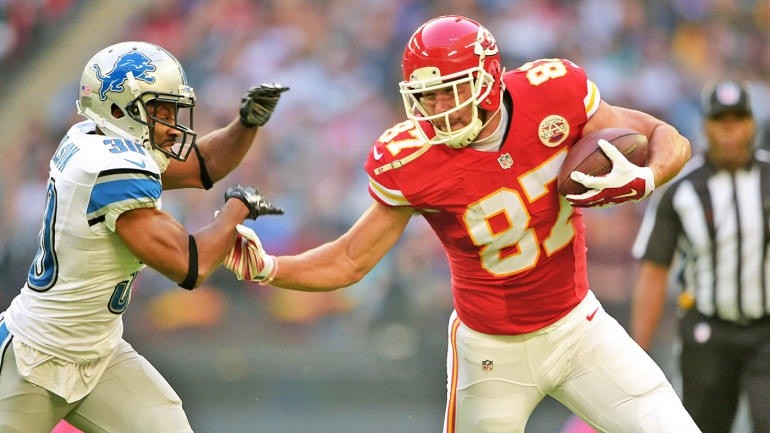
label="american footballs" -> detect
[556,128,648,194]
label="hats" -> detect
[703,81,752,117]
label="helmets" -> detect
[401,14,505,111]
[76,40,190,174]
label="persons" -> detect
[0,40,291,433]
[622,77,769,433]
[0,0,770,329]
[215,13,706,433]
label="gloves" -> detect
[566,138,654,208]
[224,182,285,220]
[213,210,278,285]
[240,82,290,127]
